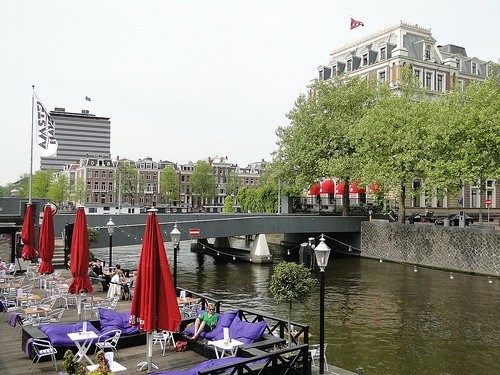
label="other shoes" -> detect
[190,335,199,340]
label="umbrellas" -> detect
[39,208,55,291]
[68,207,93,320]
[128,208,182,373]
[21,205,36,272]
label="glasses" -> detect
[209,308,215,311]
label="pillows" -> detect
[97,306,141,337]
[229,315,271,344]
[182,308,240,338]
[38,319,110,350]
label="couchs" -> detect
[173,316,288,358]
[146,345,292,375]
[21,318,146,364]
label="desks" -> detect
[177,296,202,308]
[13,294,41,306]
[123,278,131,284]
[45,277,66,295]
[102,270,114,282]
[6,275,15,282]
[209,337,245,360]
[87,360,127,375]
[67,330,99,365]
[21,308,50,322]
[86,296,106,311]
[0,282,23,293]
[57,285,68,295]
[285,330,313,346]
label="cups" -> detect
[180,295,183,300]
[224,341,229,347]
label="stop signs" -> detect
[485,198,491,205]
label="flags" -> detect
[350,18,364,29]
[86,96,92,101]
[33,91,58,157]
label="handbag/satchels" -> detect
[175,341,187,352]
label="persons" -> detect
[0,259,8,274]
[190,304,218,341]
[88,262,137,299]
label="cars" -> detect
[434,213,474,226]
[408,211,437,225]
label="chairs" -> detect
[309,342,329,372]
[0,262,200,375]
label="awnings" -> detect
[309,179,385,195]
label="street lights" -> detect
[106,215,116,273]
[170,221,181,298]
[314,235,332,375]
